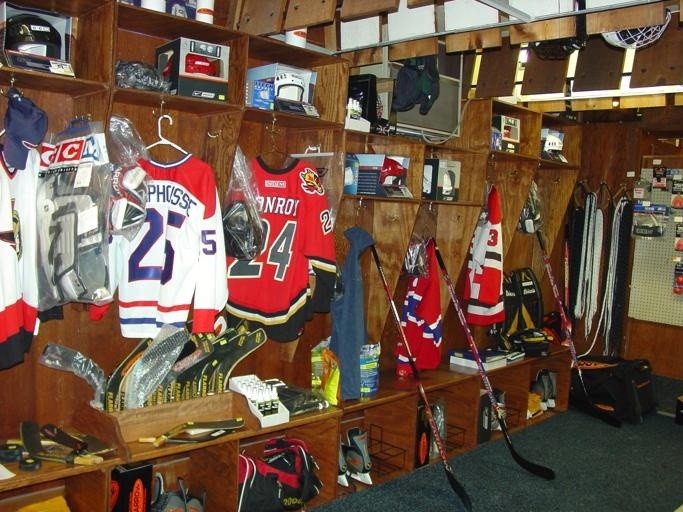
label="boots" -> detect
[344,428,371,472]
[335,435,347,474]
[529,370,556,402]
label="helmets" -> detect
[0,13,61,73]
[601,10,672,50]
[530,36,585,62]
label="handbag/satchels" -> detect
[237,435,325,512]
[571,354,659,428]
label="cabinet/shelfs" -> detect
[0,0,584,512]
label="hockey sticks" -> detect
[371,245,472,512]
[536,230,623,428]
[432,240,554,480]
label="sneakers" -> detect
[152,477,207,512]
